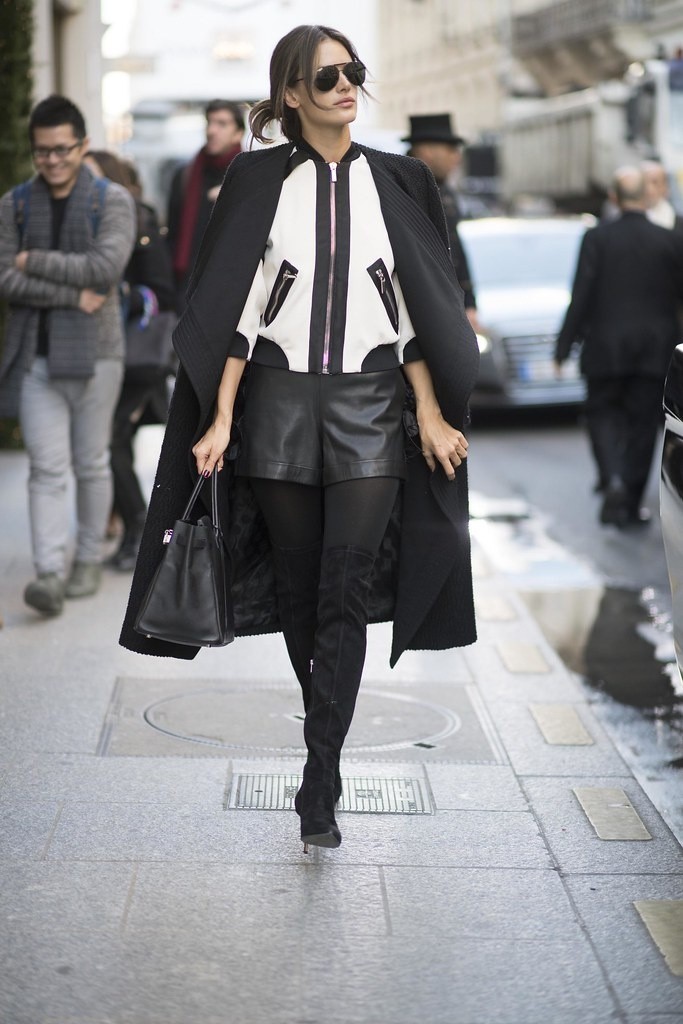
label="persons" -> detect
[166,98,249,317]
[0,92,138,615]
[190,22,471,854]
[553,156,683,529]
[76,149,176,573]
[403,110,476,324]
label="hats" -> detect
[399,114,463,145]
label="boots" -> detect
[294,545,376,850]
[272,549,342,805]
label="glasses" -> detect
[290,60,366,91]
[31,140,83,158]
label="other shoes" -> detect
[24,572,65,616]
[113,510,148,572]
[64,561,101,597]
[628,507,651,529]
[597,476,626,524]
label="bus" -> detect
[493,57,682,231]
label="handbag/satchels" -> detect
[132,461,234,647]
[121,284,178,381]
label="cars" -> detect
[456,213,594,411]
[658,344,683,679]
